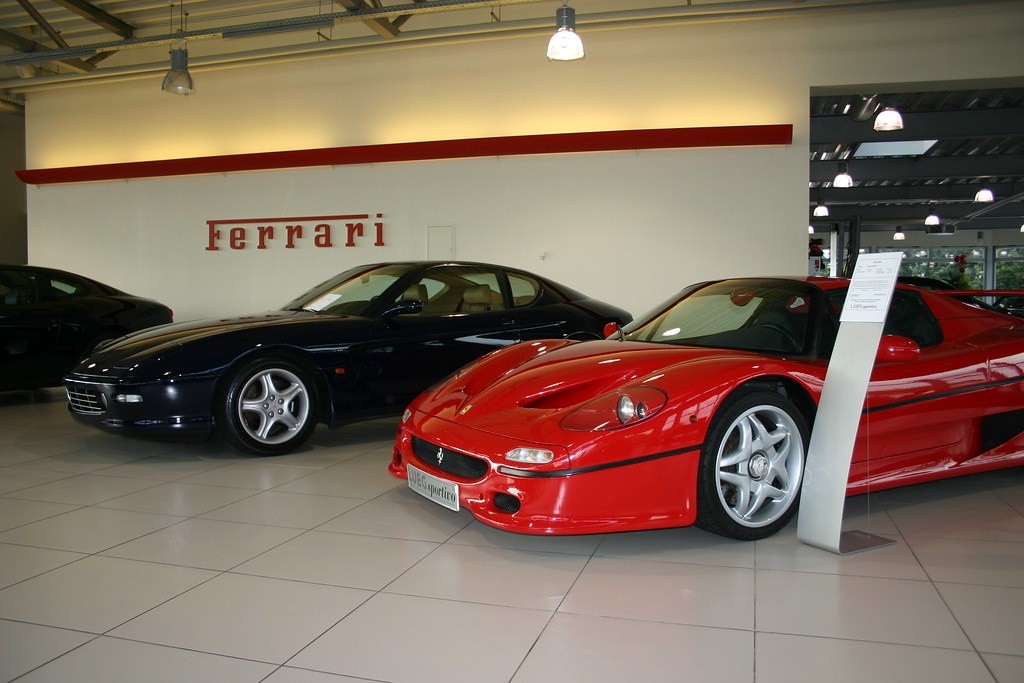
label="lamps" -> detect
[873,95,904,133]
[1019,224,1024,233]
[545,0,585,61]
[924,205,941,225]
[925,226,955,236]
[893,226,906,241]
[832,162,854,188]
[809,224,815,235]
[813,200,829,218]
[974,181,995,202]
[161,0,197,97]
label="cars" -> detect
[992,294,1024,319]
[0,264,174,391]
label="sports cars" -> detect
[388,276,1024,541]
[63,260,636,455]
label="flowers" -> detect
[936,254,975,290]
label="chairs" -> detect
[883,297,937,347]
[397,284,433,316]
[462,284,492,313]
